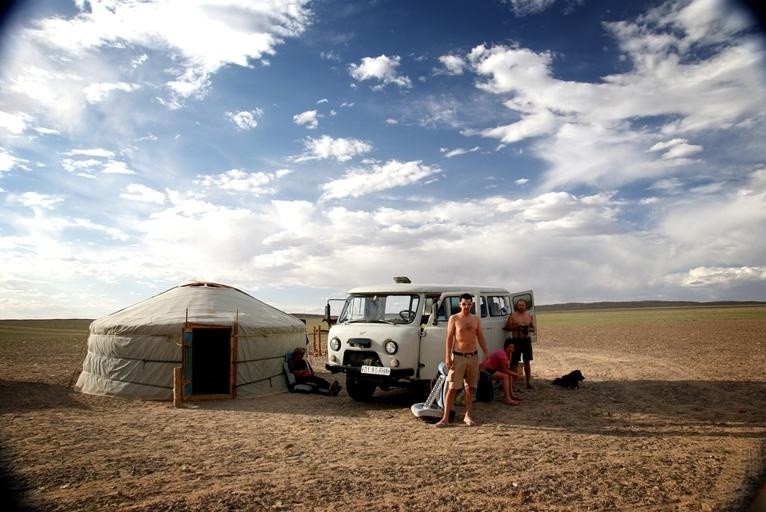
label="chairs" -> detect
[410,361,456,422]
[284,352,319,393]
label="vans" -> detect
[321,276,538,402]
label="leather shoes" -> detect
[331,381,339,393]
[333,385,342,396]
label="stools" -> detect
[475,378,496,403]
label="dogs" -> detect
[550,369,586,390]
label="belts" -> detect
[452,351,479,359]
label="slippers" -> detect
[513,396,524,400]
[505,401,519,406]
[463,419,476,426]
[436,419,448,427]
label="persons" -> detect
[479,338,527,405]
[435,294,490,428]
[287,348,342,396]
[505,299,537,392]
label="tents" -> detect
[71,281,308,400]
